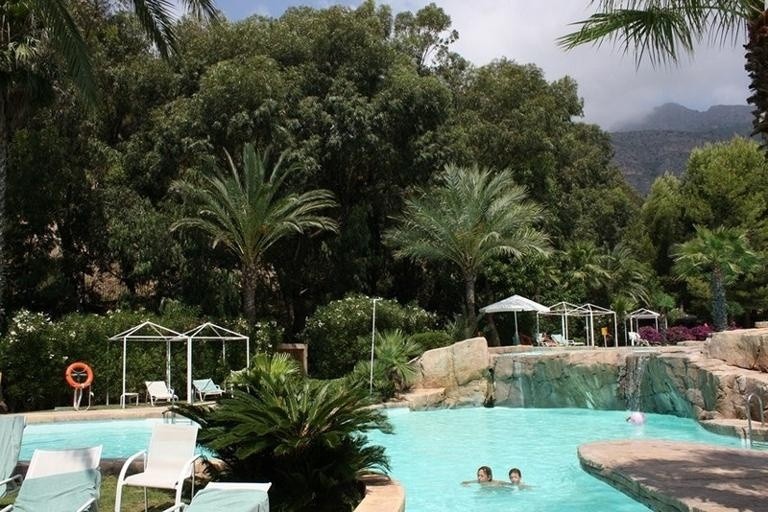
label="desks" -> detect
[120,392,139,406]
[230,383,251,398]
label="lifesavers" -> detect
[66,362,93,388]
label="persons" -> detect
[461,466,527,489]
[540,333,555,345]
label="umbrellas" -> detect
[479,294,550,342]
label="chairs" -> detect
[1,413,272,512]
[536,332,585,347]
[192,377,225,401]
[144,379,179,406]
[628,331,649,346]
